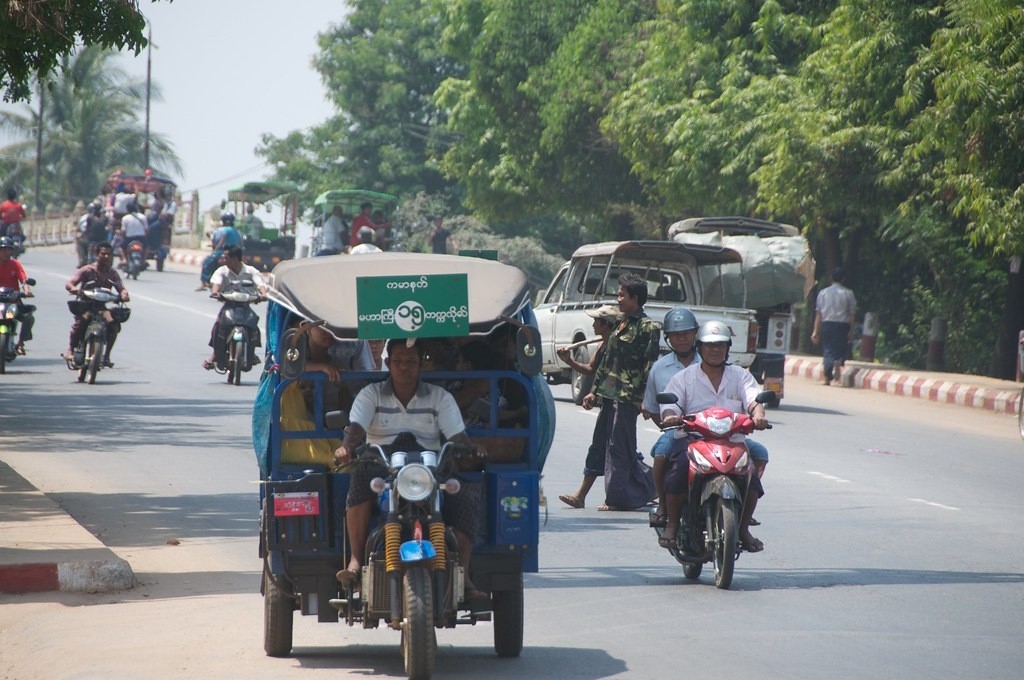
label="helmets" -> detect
[109,307,131,323]
[221,211,234,224]
[67,301,93,316]
[0,236,15,249]
[357,225,376,242]
[83,202,94,211]
[696,320,732,348]
[663,307,699,333]
[126,201,137,211]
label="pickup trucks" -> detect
[534,241,759,406]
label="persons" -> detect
[240,204,262,227]
[0,188,27,253]
[202,243,268,370]
[286,320,529,431]
[196,212,243,292]
[557,274,660,510]
[811,267,856,386]
[334,339,489,600]
[642,308,769,552]
[74,182,177,269]
[350,203,394,251]
[428,215,457,254]
[320,205,346,249]
[65,242,129,368]
[0,236,34,355]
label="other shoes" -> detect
[597,504,622,511]
[833,359,841,382]
[822,379,830,385]
[559,495,585,509]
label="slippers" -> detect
[750,517,760,527]
[202,359,213,370]
[466,587,488,600]
[742,535,764,552]
[651,506,666,526]
[659,525,677,547]
[336,568,362,593]
[15,344,26,356]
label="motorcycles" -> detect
[5,204,28,260]
[658,391,777,588]
[258,255,556,680]
[62,278,129,384]
[105,173,177,272]
[308,189,398,255]
[205,292,267,385]
[0,277,36,374]
[205,181,296,281]
[116,231,144,280]
[74,220,99,264]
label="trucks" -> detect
[669,218,794,408]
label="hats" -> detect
[585,305,621,325]
